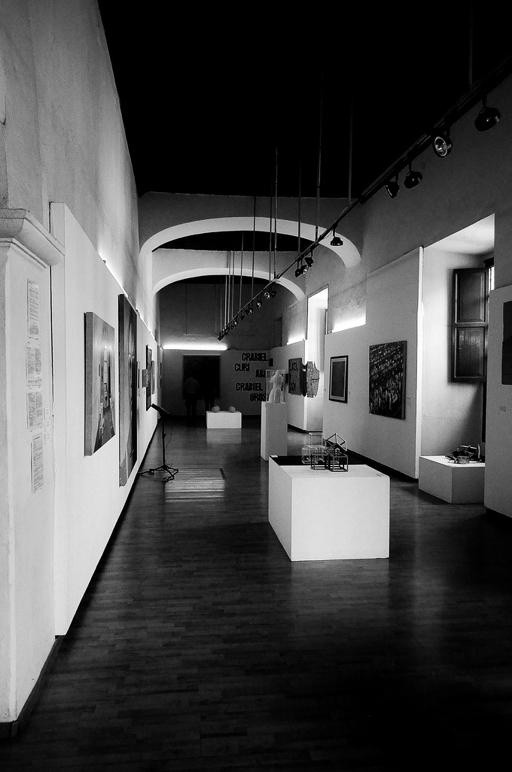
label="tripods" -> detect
[150,417,178,478]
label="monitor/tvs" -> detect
[288,358,302,395]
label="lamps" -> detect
[385,90,503,200]
[216,287,276,343]
[293,223,344,279]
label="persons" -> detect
[182,371,201,429]
[266,369,285,404]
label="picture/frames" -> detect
[328,355,348,403]
